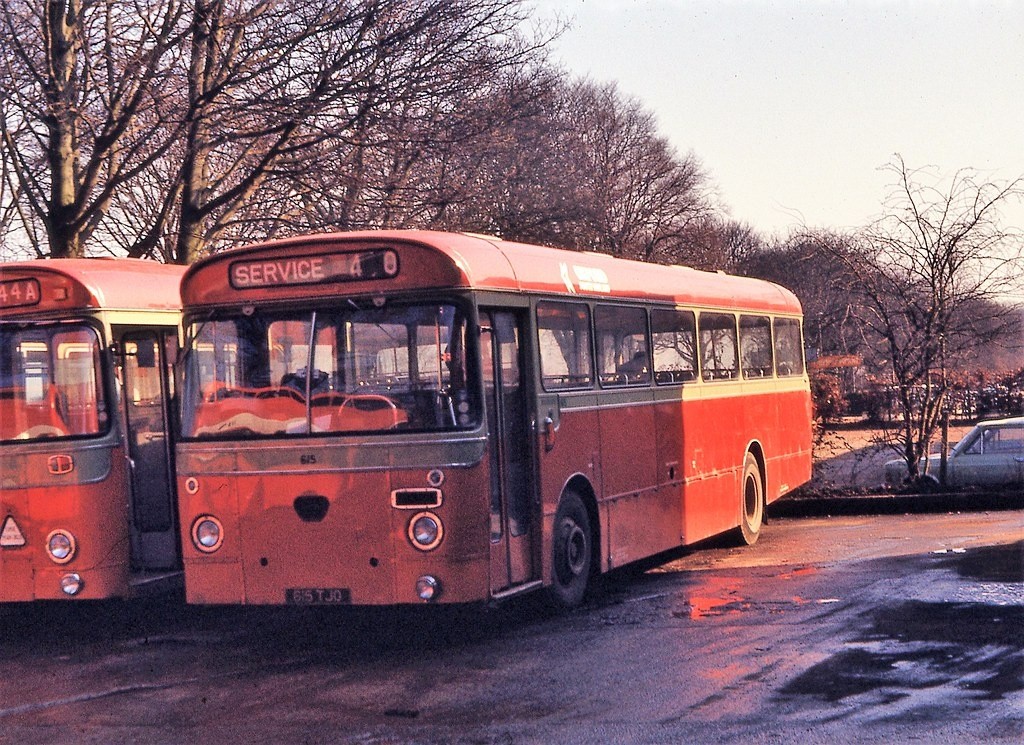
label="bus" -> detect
[0,256,617,610]
[175,229,816,618]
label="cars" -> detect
[885,416,1024,493]
